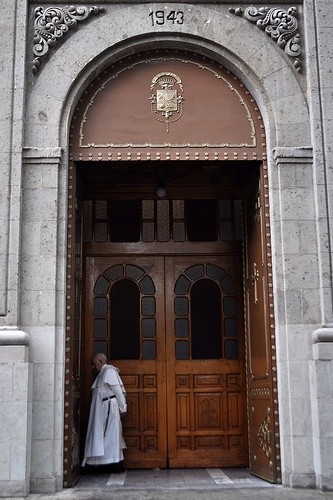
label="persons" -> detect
[81,353,129,474]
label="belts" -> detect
[102,395,115,402]
